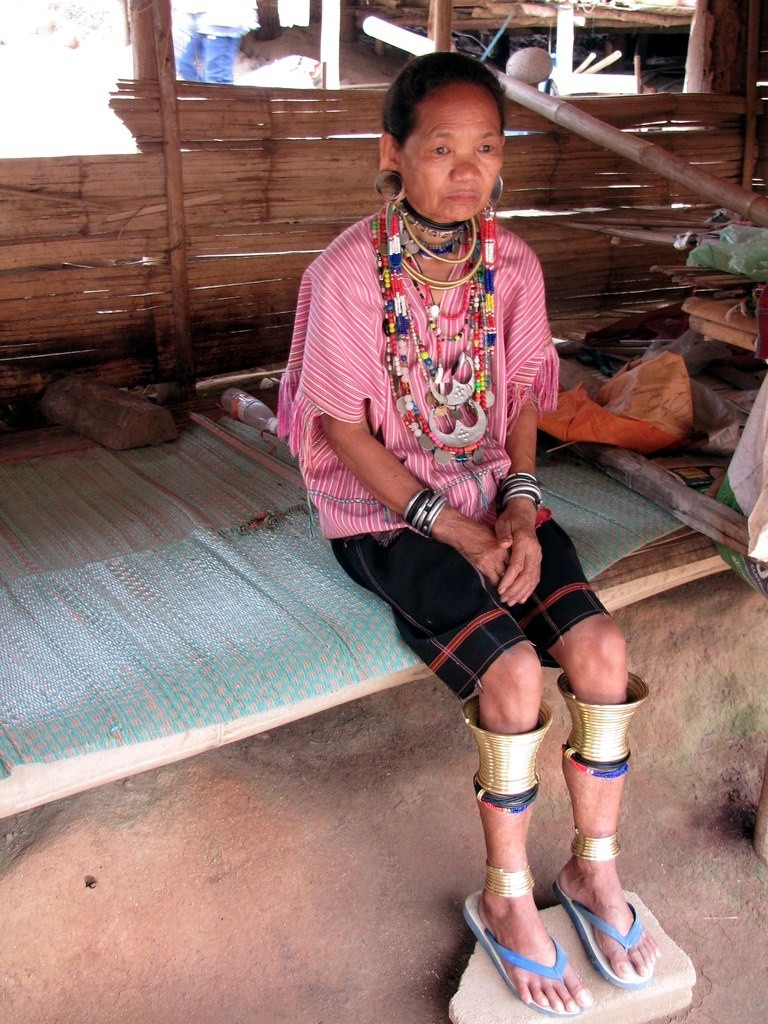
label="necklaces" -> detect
[369,186,502,467]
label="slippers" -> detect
[463,889,585,1018]
[553,878,656,990]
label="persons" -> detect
[177,0,261,87]
[274,47,667,1020]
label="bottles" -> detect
[221,387,278,434]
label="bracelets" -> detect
[497,470,544,512]
[401,486,449,539]
